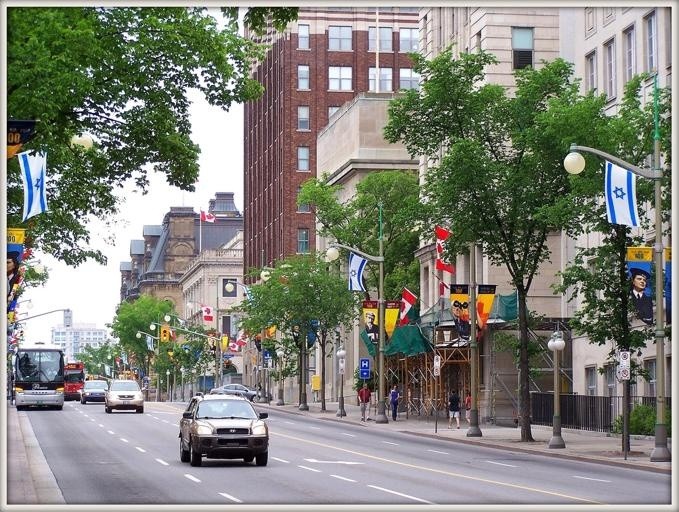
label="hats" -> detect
[366,313,375,319]
[630,268,650,279]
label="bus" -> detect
[62,360,85,401]
[15,341,65,411]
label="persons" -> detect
[366,312,388,345]
[389,385,399,420]
[447,389,461,429]
[465,393,471,423]
[358,383,372,421]
[7,251,20,294]
[630,268,652,323]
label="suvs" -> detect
[179,391,269,466]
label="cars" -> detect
[80,379,109,404]
[209,383,273,403]
[104,379,147,413]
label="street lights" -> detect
[97,313,208,403]
[28,115,102,158]
[260,264,308,412]
[546,328,566,450]
[275,349,284,407]
[326,204,389,425]
[7,298,32,403]
[335,343,346,419]
[226,278,260,404]
[564,75,671,464]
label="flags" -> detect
[17,149,48,224]
[292,320,318,349]
[146,335,155,352]
[605,160,641,227]
[348,252,368,292]
[435,225,454,273]
[202,209,215,224]
[399,287,418,326]
[450,283,497,337]
